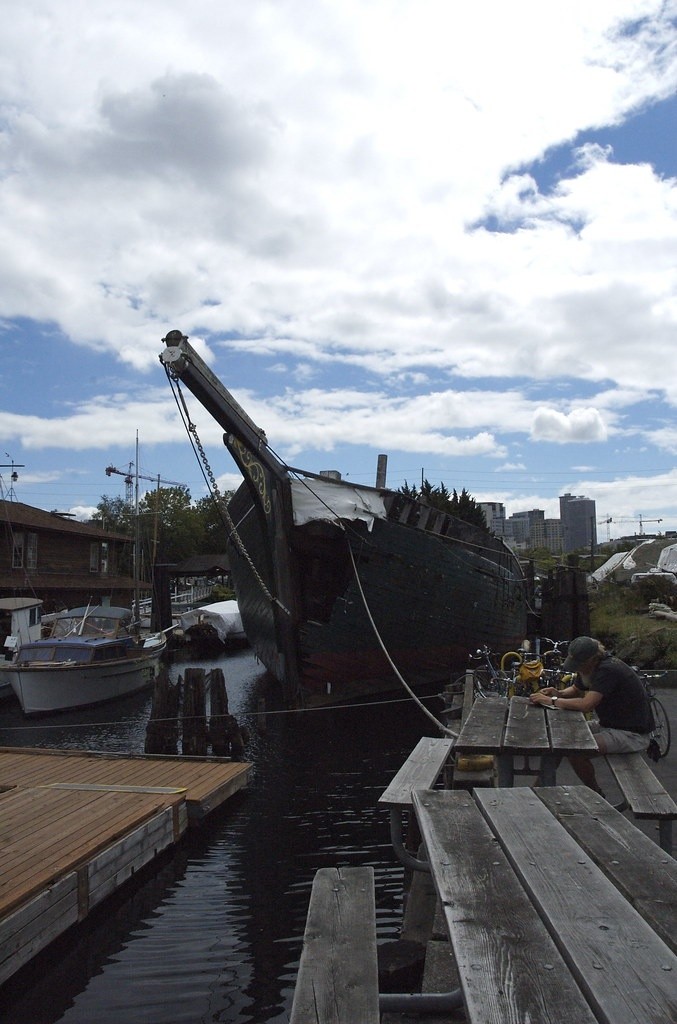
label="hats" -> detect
[563,636,598,671]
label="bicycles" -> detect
[467,638,670,759]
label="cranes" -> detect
[106,429,188,534]
[598,518,613,542]
[613,514,663,534]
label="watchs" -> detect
[551,696,557,707]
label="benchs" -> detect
[289,867,450,1024]
[376,736,455,873]
[604,753,677,856]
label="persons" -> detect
[529,636,656,799]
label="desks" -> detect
[410,783,677,1024]
[454,697,600,788]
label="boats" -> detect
[157,328,529,707]
[0,598,75,698]
[179,599,249,644]
[0,606,169,713]
